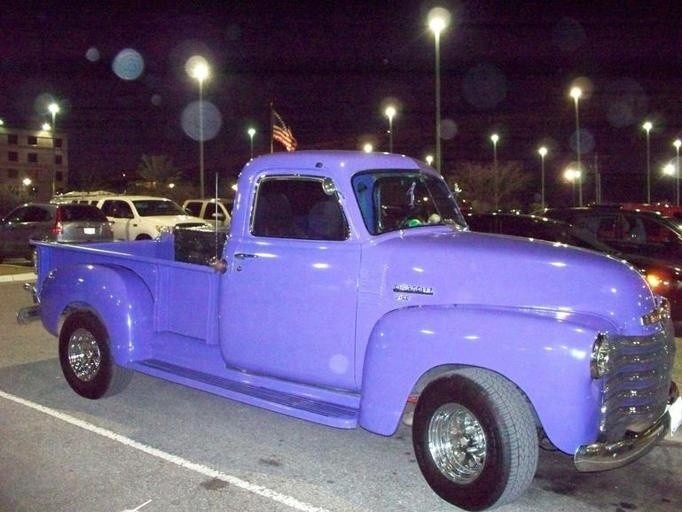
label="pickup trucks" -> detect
[15,150,682,512]
[55,195,213,241]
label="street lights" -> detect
[190,58,209,197]
[570,86,586,207]
[385,102,396,154]
[673,139,682,207]
[643,121,653,204]
[47,101,59,194]
[539,146,549,209]
[246,128,258,158]
[425,155,433,166]
[429,15,446,173]
[490,133,502,210]
[566,167,579,207]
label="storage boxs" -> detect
[171,221,226,270]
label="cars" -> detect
[0,201,114,265]
[461,197,682,327]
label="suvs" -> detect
[183,197,234,228]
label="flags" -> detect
[271,109,299,152]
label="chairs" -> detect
[255,193,307,239]
[143,202,162,217]
